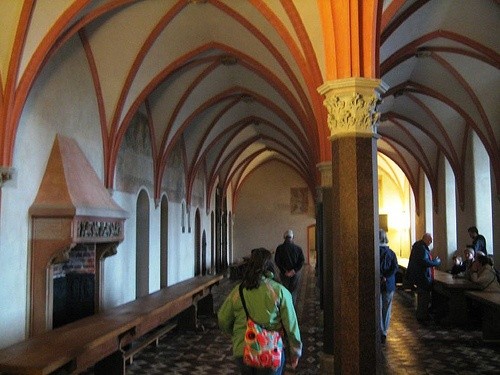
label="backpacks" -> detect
[496,270,500,284]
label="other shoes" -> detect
[381,334,386,344]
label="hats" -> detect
[283,229,294,239]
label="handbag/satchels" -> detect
[242,317,284,371]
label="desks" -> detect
[0,273,223,374]
[397,259,470,309]
[461,289,500,320]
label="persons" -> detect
[404,233,442,323]
[451,226,500,292]
[275,229,304,307]
[379,228,399,344]
[216,247,303,375]
